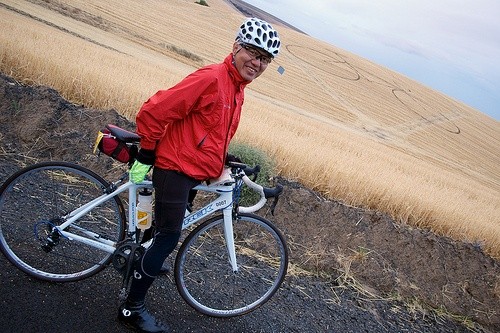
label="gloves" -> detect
[224,153,242,168]
[136,149,156,173]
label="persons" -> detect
[117,19,281,333]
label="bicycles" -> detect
[0,123,288,318]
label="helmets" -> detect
[235,17,281,59]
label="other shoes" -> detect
[159,263,171,276]
[117,304,167,333]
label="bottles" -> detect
[136,187,153,230]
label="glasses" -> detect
[240,44,272,64]
